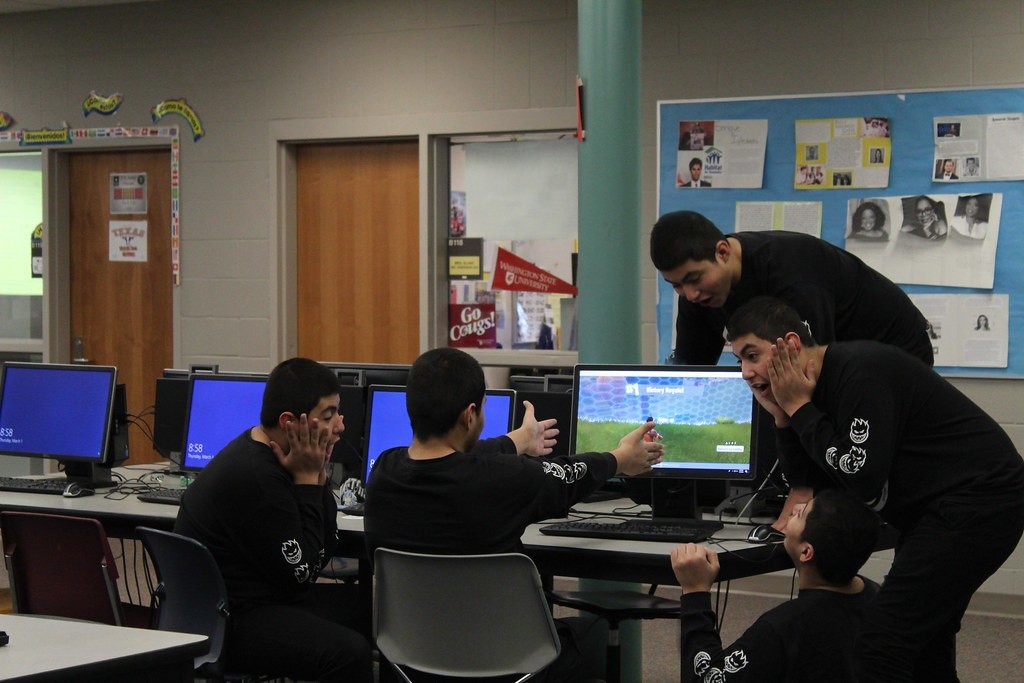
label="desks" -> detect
[0,613,209,683]
[0,463,896,642]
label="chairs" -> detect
[1,510,127,629]
[368,544,561,683]
[548,584,685,683]
[132,524,230,675]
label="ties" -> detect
[695,183,698,188]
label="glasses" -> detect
[915,207,934,217]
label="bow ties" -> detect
[945,173,951,177]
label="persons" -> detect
[935,159,958,179]
[901,197,946,240]
[949,124,960,136]
[873,149,884,163]
[680,157,712,187]
[796,167,824,185]
[952,196,989,238]
[646,416,663,442]
[649,210,931,372]
[963,158,980,176]
[974,314,991,332]
[724,297,1024,683]
[668,490,914,683]
[847,203,888,242]
[362,347,656,683]
[806,147,817,160]
[833,174,850,186]
[170,354,375,682]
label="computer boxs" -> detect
[58,383,130,465]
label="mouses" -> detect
[63,482,82,497]
[748,525,786,545]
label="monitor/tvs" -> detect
[0,361,118,491]
[153,364,759,521]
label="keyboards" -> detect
[540,521,724,544]
[0,476,75,495]
[138,491,185,506]
